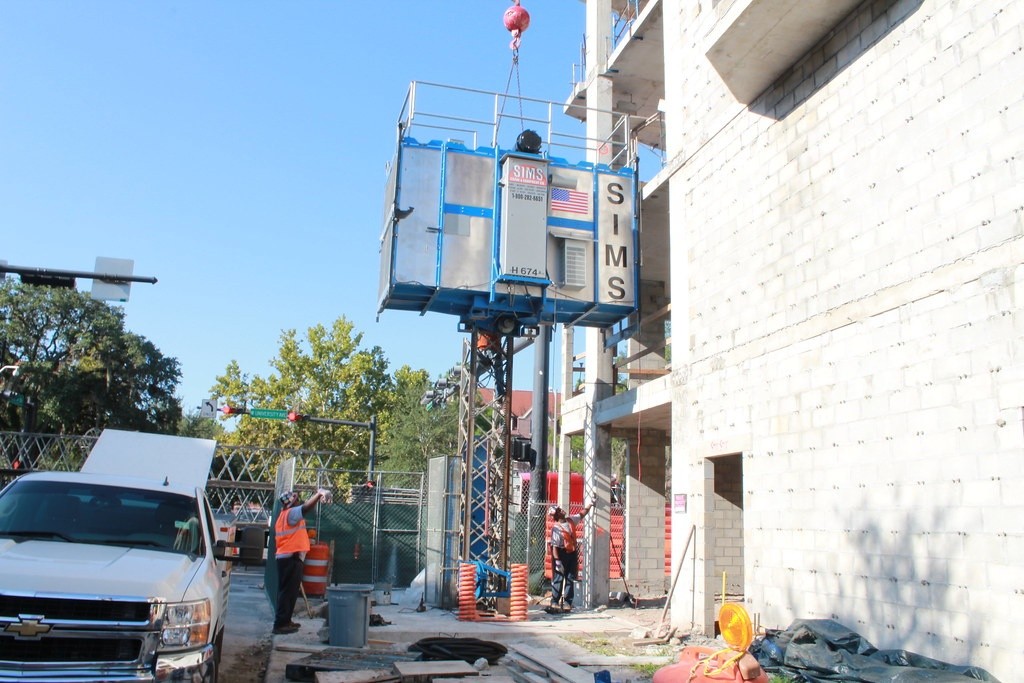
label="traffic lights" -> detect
[289,413,310,423]
[222,405,244,414]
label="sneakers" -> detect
[271,622,302,634]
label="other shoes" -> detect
[551,603,571,612]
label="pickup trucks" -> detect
[1,428,242,683]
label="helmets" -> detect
[548,505,560,518]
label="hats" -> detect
[278,491,297,509]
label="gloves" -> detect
[554,560,565,575]
[590,493,599,506]
[318,487,330,502]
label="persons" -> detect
[546,492,598,610]
[275,489,333,634]
